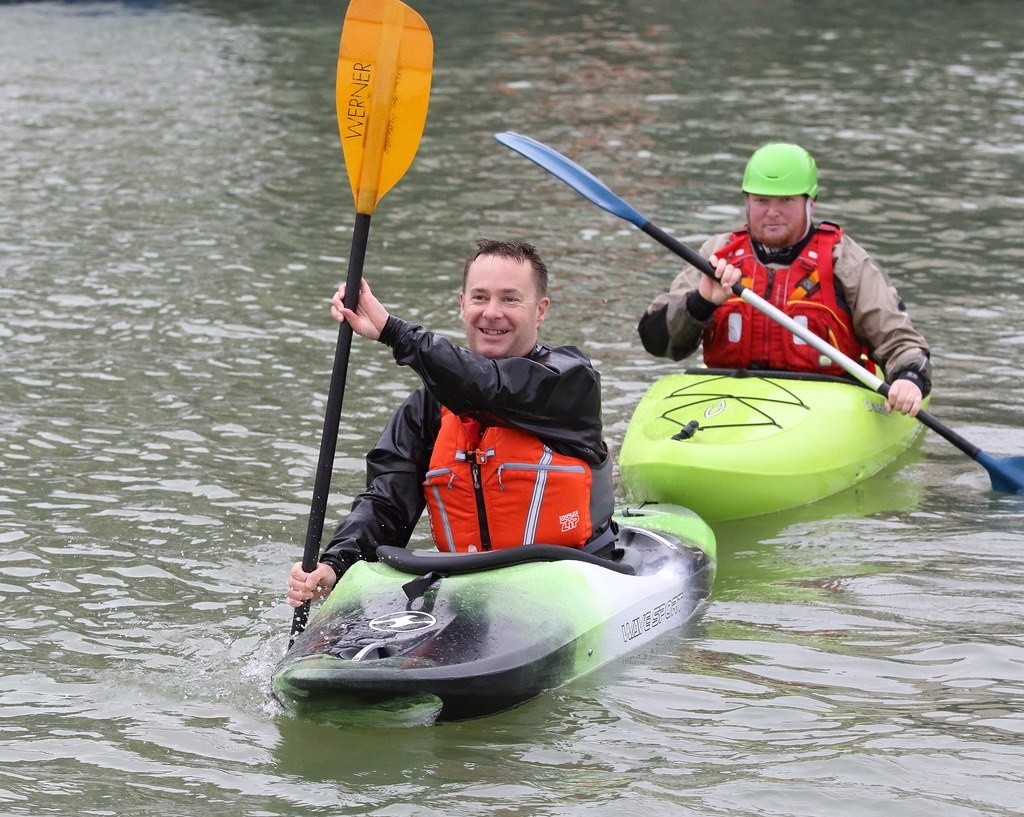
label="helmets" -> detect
[740,141,819,198]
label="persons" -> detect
[638,142,933,418]
[286,237,618,608]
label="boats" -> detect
[269,502,716,724]
[619,366,935,523]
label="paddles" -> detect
[495,131,1023,496]
[287,0,435,654]
